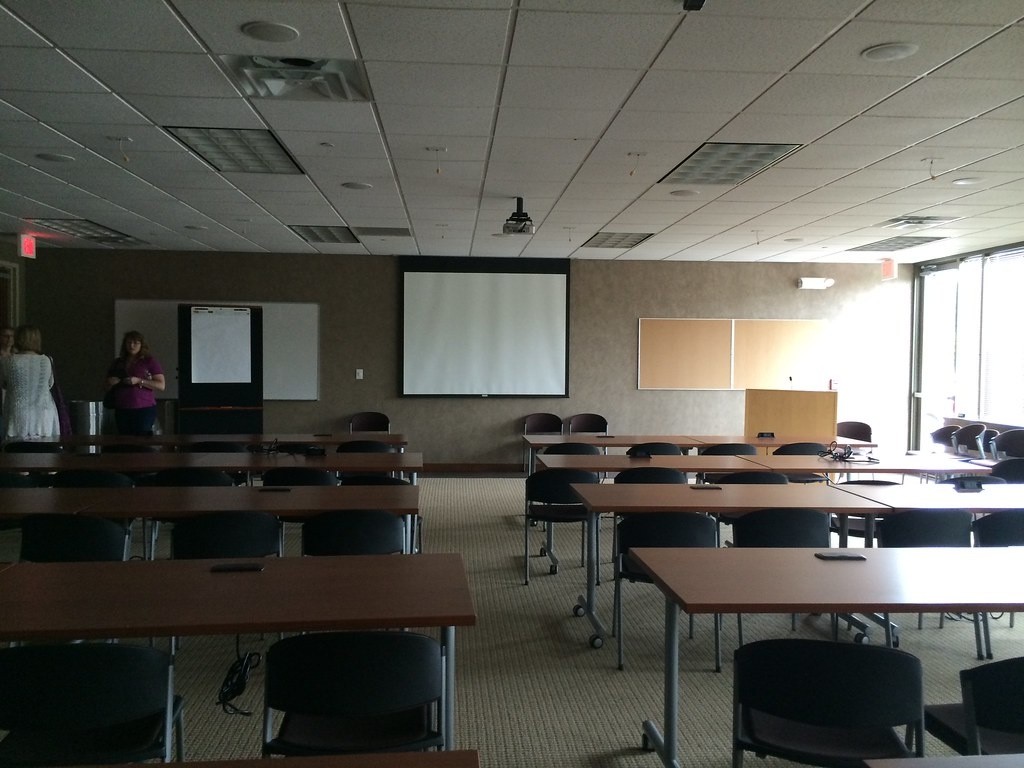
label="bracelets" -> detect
[140,378,143,389]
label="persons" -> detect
[104,330,166,436]
[0,325,61,489]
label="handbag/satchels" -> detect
[51,385,74,436]
[102,382,121,409]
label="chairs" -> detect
[520,410,1024,768]
[348,411,392,436]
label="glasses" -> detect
[126,340,141,345]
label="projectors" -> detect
[503,221,535,234]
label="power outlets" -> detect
[355,368,364,380]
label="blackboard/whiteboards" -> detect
[112,295,323,404]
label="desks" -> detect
[0,426,482,766]
[742,386,838,439]
